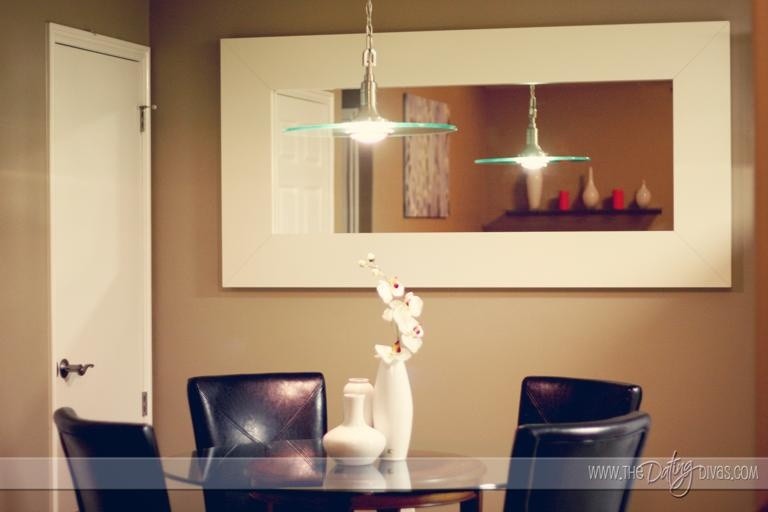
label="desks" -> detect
[160,439,486,511]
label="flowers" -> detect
[355,252,426,366]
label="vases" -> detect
[372,356,413,461]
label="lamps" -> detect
[281,1,460,145]
[473,83,592,170]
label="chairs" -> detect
[54,407,171,512]
[505,376,651,511]
[186,372,351,510]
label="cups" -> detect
[559,190,571,211]
[611,187,625,210]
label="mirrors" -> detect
[216,18,734,291]
